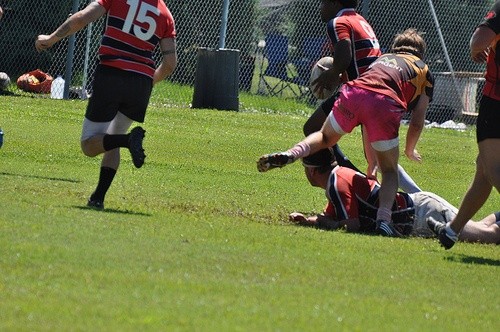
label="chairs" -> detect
[258,34,328,105]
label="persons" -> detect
[303,0,423,193]
[427,0,500,249]
[257,28,435,238]
[289,147,500,243]
[36,0,176,208]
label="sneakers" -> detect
[87,192,104,210]
[374,219,403,238]
[256,152,294,172]
[128,126,146,168]
[425,216,458,250]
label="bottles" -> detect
[51,75,65,99]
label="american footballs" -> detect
[310,56,342,100]
